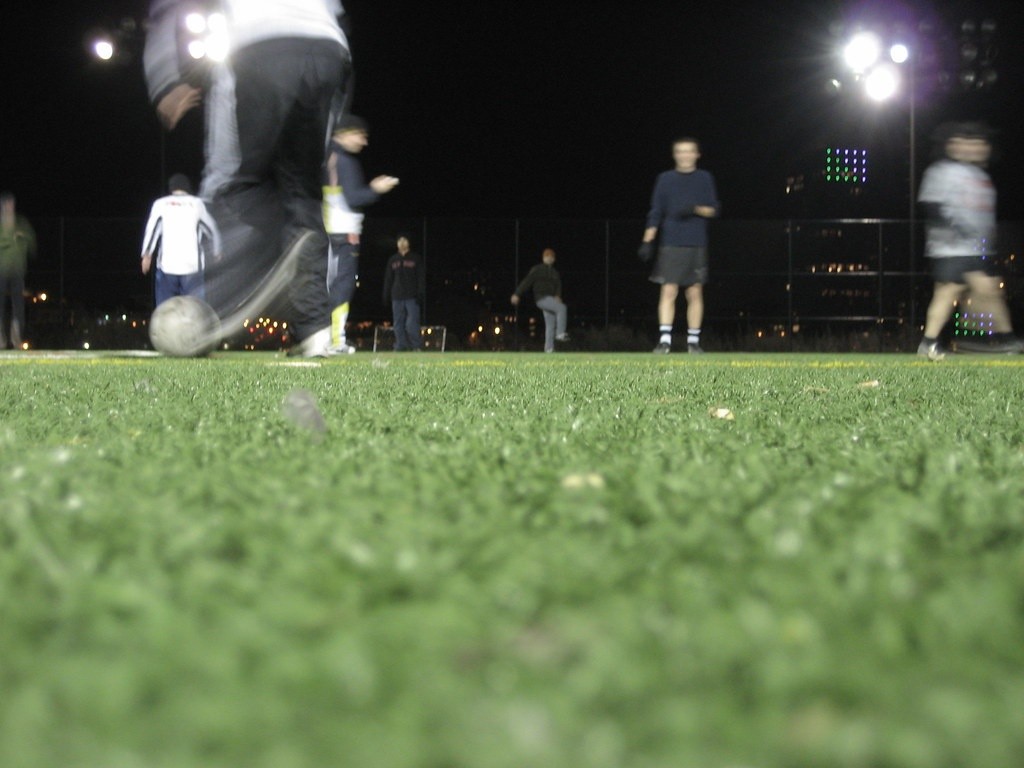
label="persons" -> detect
[379,236,426,350]
[637,137,720,352]
[512,247,569,354]
[0,192,37,349]
[142,0,355,356]
[913,125,1024,360]
[315,118,400,354]
[141,173,222,307]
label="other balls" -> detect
[147,295,221,358]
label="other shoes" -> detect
[555,335,571,343]
[917,340,950,361]
[186,232,325,342]
[687,342,703,355]
[286,328,332,358]
[653,342,670,355]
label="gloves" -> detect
[637,243,652,266]
[677,203,696,222]
